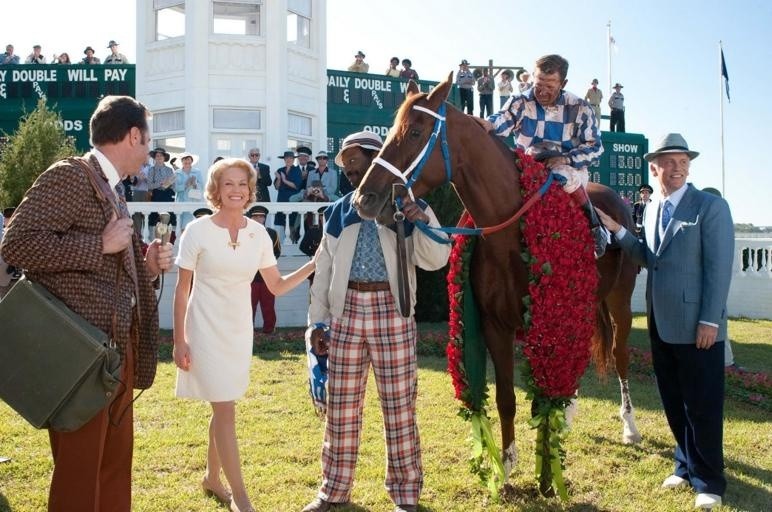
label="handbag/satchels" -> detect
[0,274,122,435]
[145,191,152,201]
[187,183,201,199]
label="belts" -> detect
[348,280,390,292]
[591,104,599,108]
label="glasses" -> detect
[251,153,258,156]
[318,156,327,160]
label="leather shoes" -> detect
[395,505,417,512]
[301,497,331,512]
[695,493,722,509]
[661,475,689,489]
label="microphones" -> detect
[157,213,173,247]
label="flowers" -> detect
[444,151,602,512]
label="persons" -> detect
[172,159,316,511]
[347,50,421,81]
[1,207,24,299]
[590,133,735,510]
[456,59,533,121]
[305,128,453,512]
[1,96,173,512]
[123,146,355,258]
[584,78,627,132]
[1,39,130,66]
[628,184,653,242]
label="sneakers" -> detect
[592,225,608,259]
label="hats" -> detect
[277,147,328,167]
[193,208,213,218]
[355,50,365,58]
[335,131,383,168]
[149,148,170,161]
[643,132,700,162]
[249,206,268,216]
[32,40,119,54]
[459,59,623,88]
[639,184,653,193]
[173,152,199,169]
[318,205,328,214]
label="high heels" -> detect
[230,498,255,512]
[200,475,232,503]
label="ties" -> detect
[302,166,304,171]
[662,199,671,232]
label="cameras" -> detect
[312,187,320,194]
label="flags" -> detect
[610,26,619,60]
[722,46,731,102]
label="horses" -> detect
[352,70,639,482]
